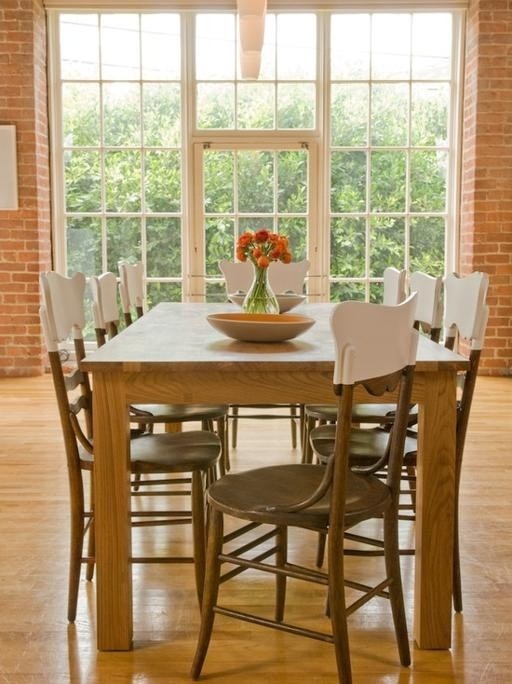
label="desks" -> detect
[80,300,454,649]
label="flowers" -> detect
[236,227,292,313]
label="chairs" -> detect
[189,290,421,679]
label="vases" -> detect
[240,262,281,315]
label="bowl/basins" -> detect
[204,310,317,343]
[225,293,306,311]
